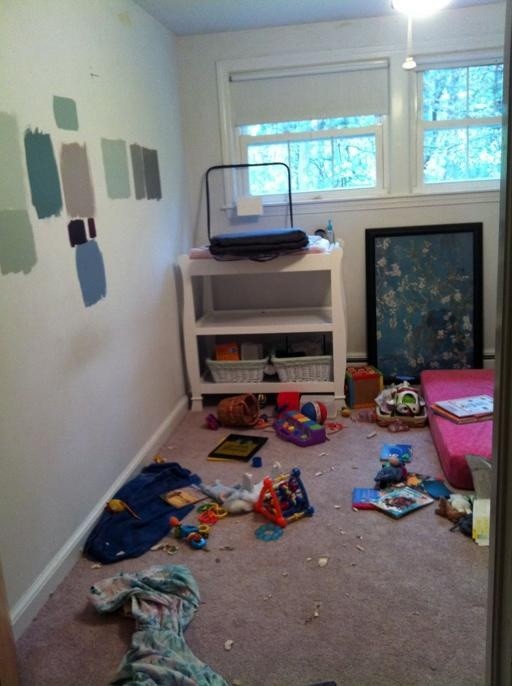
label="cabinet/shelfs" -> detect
[177,242,348,411]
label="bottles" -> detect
[326,220,335,244]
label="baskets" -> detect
[205,350,270,383]
[270,341,333,381]
[376,406,427,428]
[218,395,261,428]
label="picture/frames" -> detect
[364,221,484,386]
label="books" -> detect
[429,403,494,424]
[434,392,494,418]
[374,485,433,519]
[207,433,268,464]
